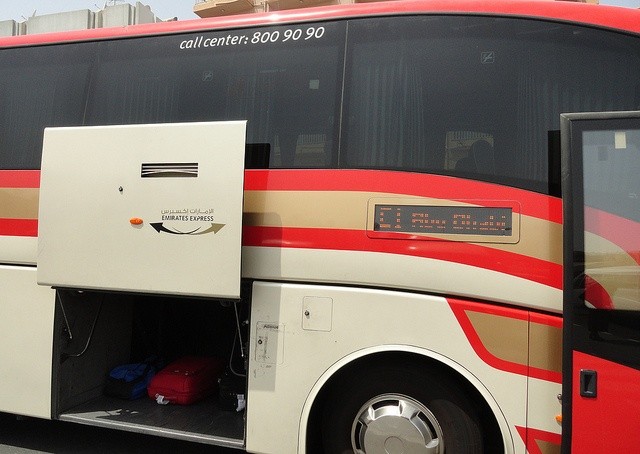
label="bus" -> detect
[0,1,640,454]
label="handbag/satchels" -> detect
[104,362,155,399]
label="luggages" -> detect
[150,353,215,406]
